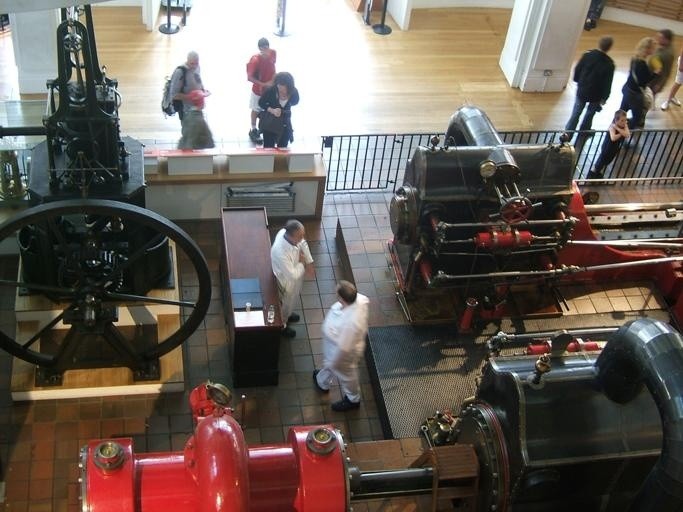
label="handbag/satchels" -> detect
[643,86,654,109]
[259,108,284,135]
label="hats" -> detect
[189,89,206,103]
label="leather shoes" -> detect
[282,326,296,337]
[331,395,360,411]
[249,129,263,144]
[288,312,299,322]
[313,369,329,393]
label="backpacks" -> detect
[161,66,186,114]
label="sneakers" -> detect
[671,97,681,106]
[661,100,670,110]
[587,129,595,135]
[589,169,604,178]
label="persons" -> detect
[271,220,313,338]
[584,0,607,31]
[312,279,371,412]
[170,49,206,127]
[620,37,657,128]
[646,29,675,112]
[246,37,279,142]
[177,89,215,151]
[662,48,683,110]
[561,36,616,147]
[257,72,300,149]
[592,110,630,173]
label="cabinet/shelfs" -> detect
[220,206,284,387]
[146,151,327,219]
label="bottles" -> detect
[244,302,251,312]
[266,304,275,324]
